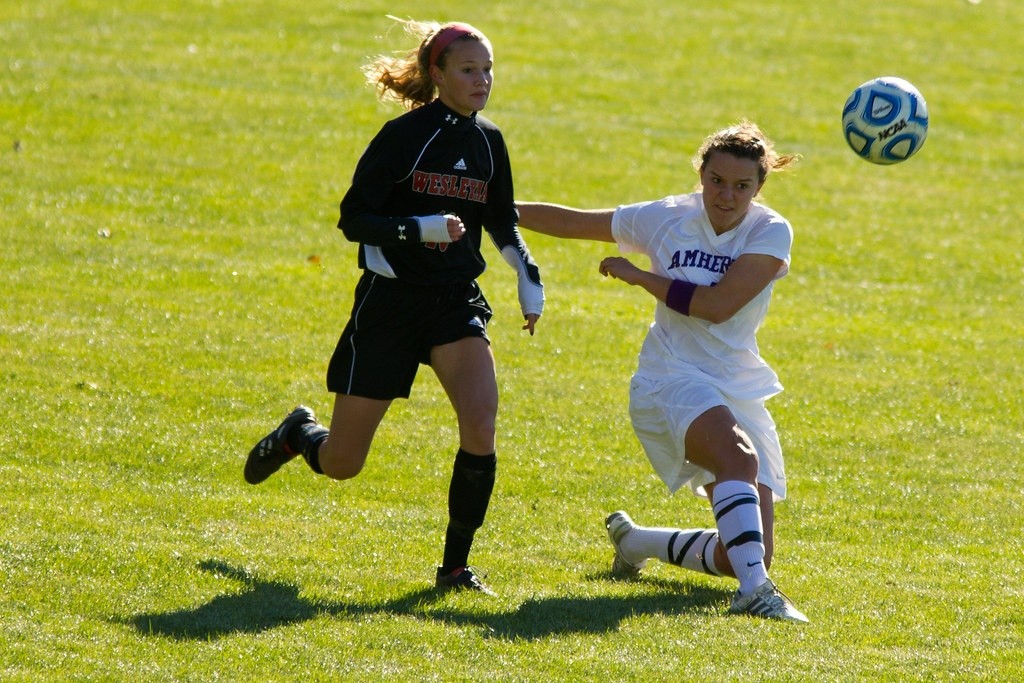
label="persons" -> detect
[242,22,545,596]
[514,120,813,624]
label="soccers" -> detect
[840,75,932,168]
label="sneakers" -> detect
[605,511,648,578]
[731,578,810,624]
[436,565,497,598]
[244,406,316,485]
